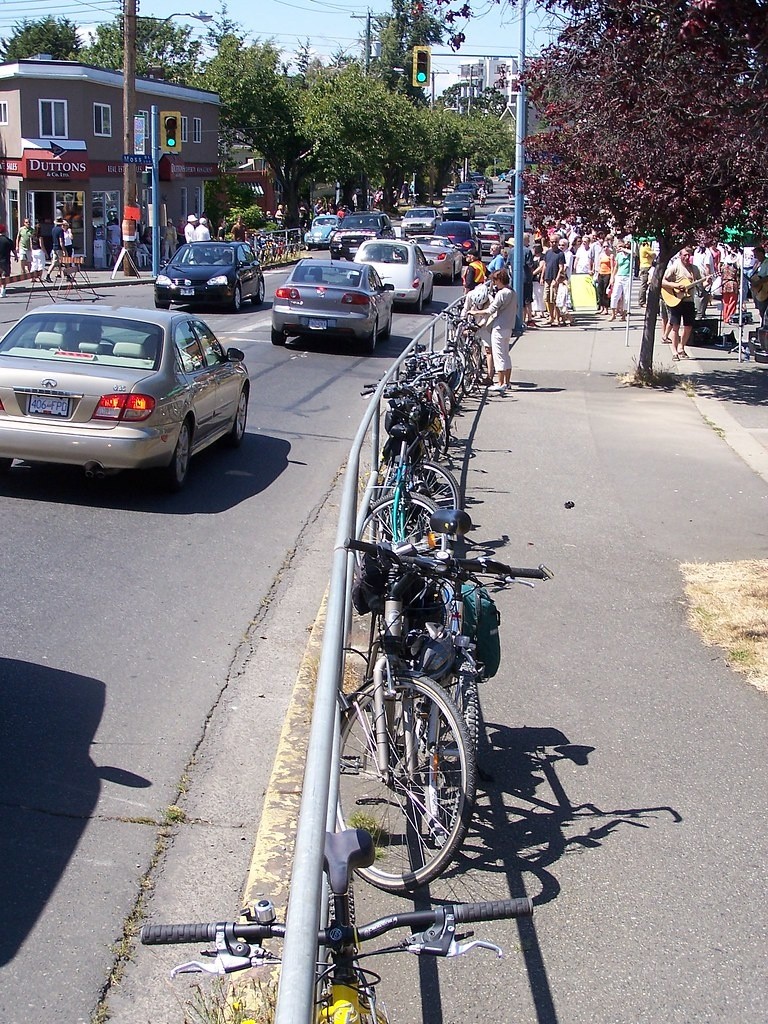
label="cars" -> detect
[269,260,394,355]
[407,234,464,286]
[0,301,250,495]
[433,221,484,266]
[353,239,434,314]
[154,240,266,313]
[399,207,444,241]
[441,171,536,254]
[304,215,344,251]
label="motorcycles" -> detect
[478,192,487,208]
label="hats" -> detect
[167,218,172,223]
[464,248,479,257]
[505,237,514,246]
[54,216,67,225]
[187,214,198,222]
[56,202,64,206]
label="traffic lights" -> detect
[159,111,181,152]
[413,46,430,87]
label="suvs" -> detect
[329,213,396,261]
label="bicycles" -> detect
[321,297,556,894]
[140,825,535,1024]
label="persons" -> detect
[16,218,34,273]
[352,188,397,211]
[184,214,212,244]
[462,216,768,361]
[30,223,48,282]
[0,223,19,298]
[466,269,517,391]
[459,285,495,386]
[161,218,179,267]
[276,205,282,224]
[217,218,226,241]
[107,217,120,252]
[231,217,246,241]
[299,199,352,233]
[402,181,409,204]
[56,201,64,219]
[478,185,488,205]
[44,216,77,283]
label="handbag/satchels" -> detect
[444,584,502,678]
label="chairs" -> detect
[35,331,66,350]
[78,342,104,354]
[113,340,143,359]
[342,278,356,286]
[303,275,317,284]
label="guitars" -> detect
[661,270,725,308]
[743,273,768,303]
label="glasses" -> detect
[558,244,565,246]
[603,246,609,248]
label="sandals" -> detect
[661,338,672,344]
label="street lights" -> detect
[122,11,213,275]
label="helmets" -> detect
[469,284,488,304]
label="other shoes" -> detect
[596,307,626,322]
[44,277,53,283]
[482,377,494,385]
[679,351,689,359]
[56,275,61,277]
[67,278,76,282]
[489,383,511,390]
[672,355,680,361]
[526,317,575,327]
[1,290,6,298]
